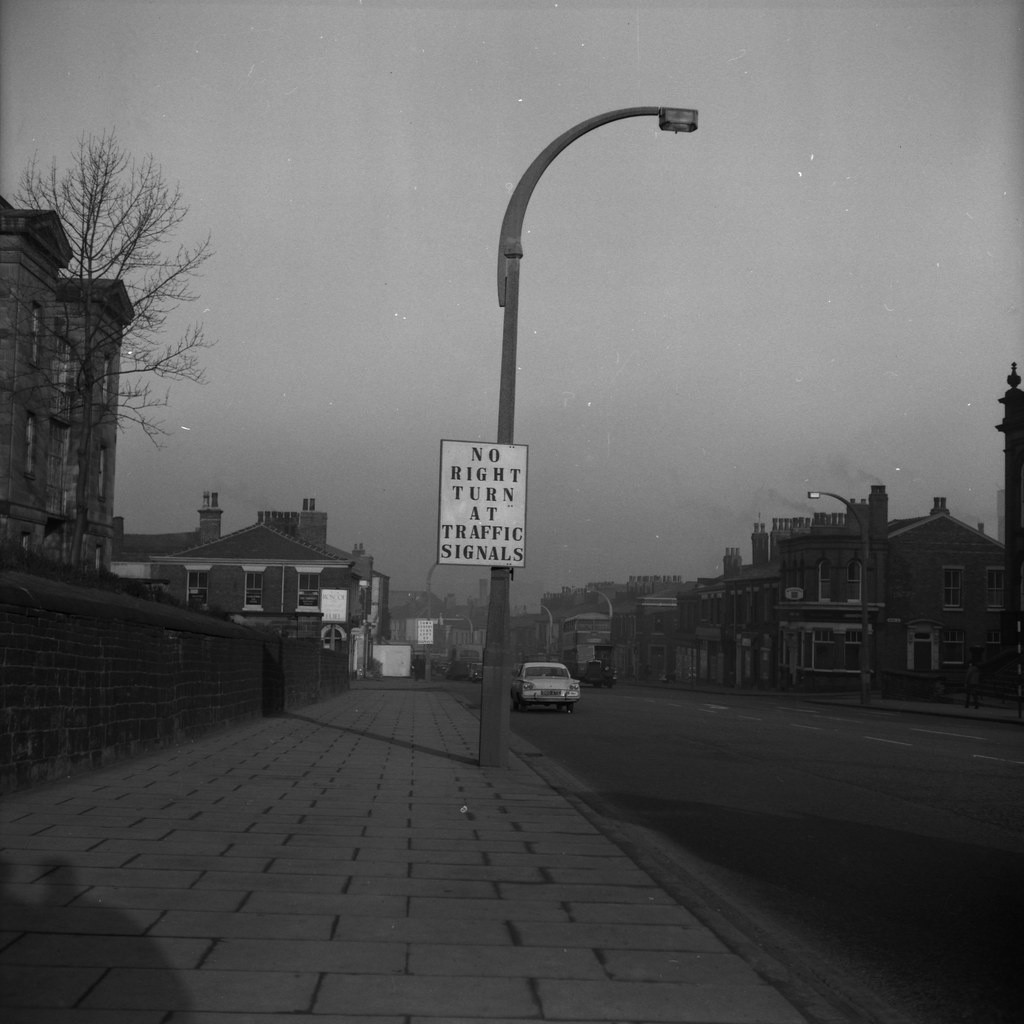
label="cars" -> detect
[431,657,483,683]
[511,662,581,713]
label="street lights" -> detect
[808,490,871,705]
[517,602,553,662]
[571,585,614,689]
[479,102,700,769]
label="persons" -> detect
[962,660,980,708]
